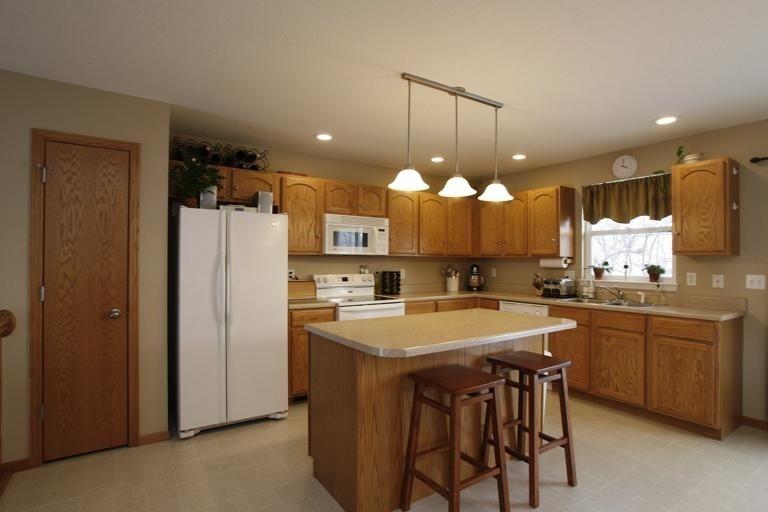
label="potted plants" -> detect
[640,263,666,282]
[584,259,614,280]
[169,159,221,209]
[674,145,702,164]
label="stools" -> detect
[477,349,577,508]
[398,362,512,511]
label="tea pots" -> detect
[467,274,484,291]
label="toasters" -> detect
[559,278,576,296]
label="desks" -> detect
[305,306,579,511]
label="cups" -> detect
[446,278,460,292]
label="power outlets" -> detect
[743,273,768,292]
[685,270,697,288]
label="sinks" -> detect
[603,299,658,308]
[560,297,616,305]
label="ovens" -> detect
[336,302,407,322]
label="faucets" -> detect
[599,285,619,297]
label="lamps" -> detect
[385,73,517,203]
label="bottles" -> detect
[360,264,368,274]
[542,280,560,296]
[381,271,400,295]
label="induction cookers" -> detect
[325,293,398,304]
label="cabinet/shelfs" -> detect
[419,191,472,258]
[405,296,464,316]
[526,184,576,259]
[289,305,337,403]
[545,305,592,401]
[647,307,747,443]
[473,189,527,259]
[591,309,645,418]
[387,188,419,256]
[172,161,280,206]
[671,158,740,258]
[280,173,320,255]
[323,180,388,217]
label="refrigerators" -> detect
[169,206,292,438]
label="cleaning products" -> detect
[624,264,629,280]
[637,290,646,303]
[655,282,662,304]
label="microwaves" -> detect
[321,213,389,258]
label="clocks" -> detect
[610,153,640,180]
